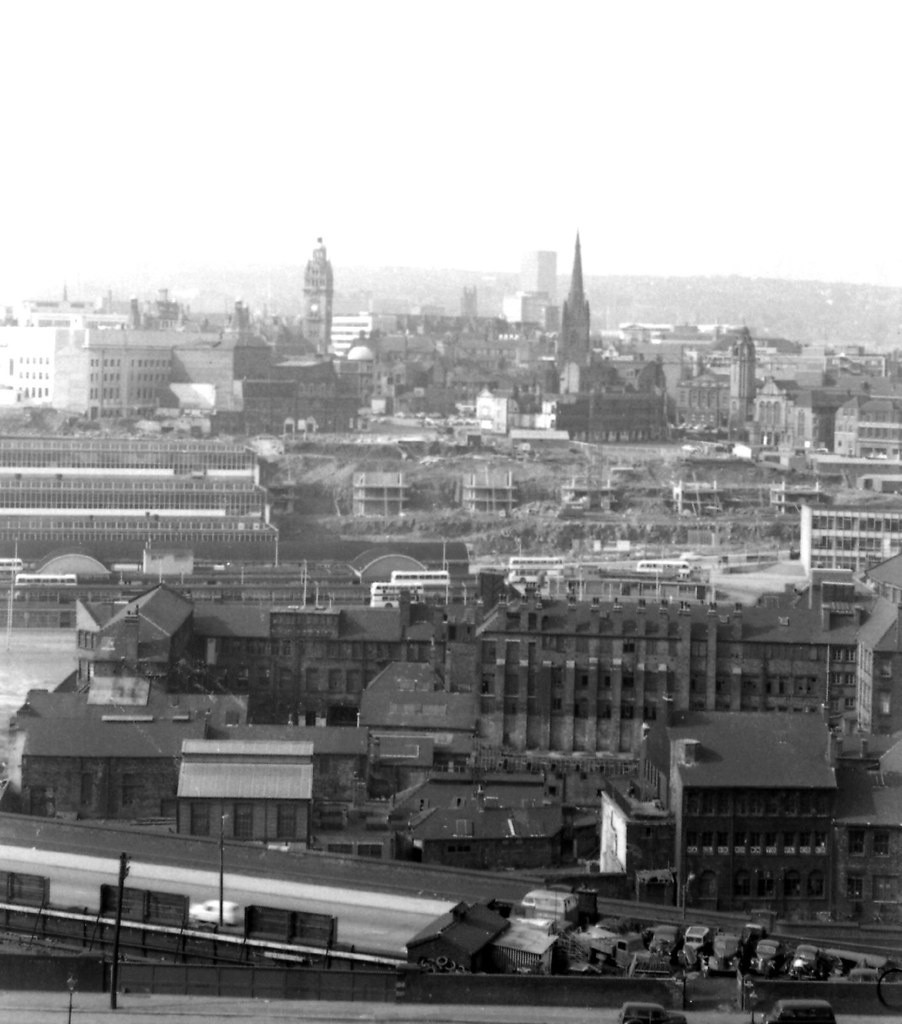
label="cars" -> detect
[618,1002,687,1024]
[749,938,794,978]
[762,999,836,1024]
[596,918,681,956]
[791,944,834,981]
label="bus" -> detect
[635,560,694,576]
[371,582,427,607]
[391,570,450,586]
[510,555,564,570]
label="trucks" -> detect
[708,926,752,974]
[576,926,661,969]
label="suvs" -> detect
[677,926,710,964]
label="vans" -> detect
[523,888,577,912]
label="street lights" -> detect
[678,873,695,920]
